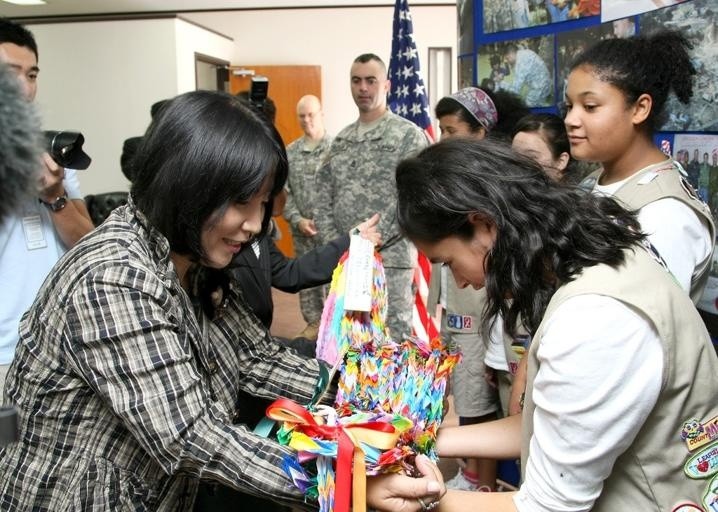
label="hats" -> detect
[444,87,497,133]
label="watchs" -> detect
[40,187,69,212]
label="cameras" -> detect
[250,75,270,110]
[30,131,94,170]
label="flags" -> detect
[386,1,438,147]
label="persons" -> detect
[1,90,448,512]
[311,52,434,347]
[1,17,98,512]
[395,27,718,512]
[281,92,345,331]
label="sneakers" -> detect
[444,468,476,491]
[294,323,321,341]
